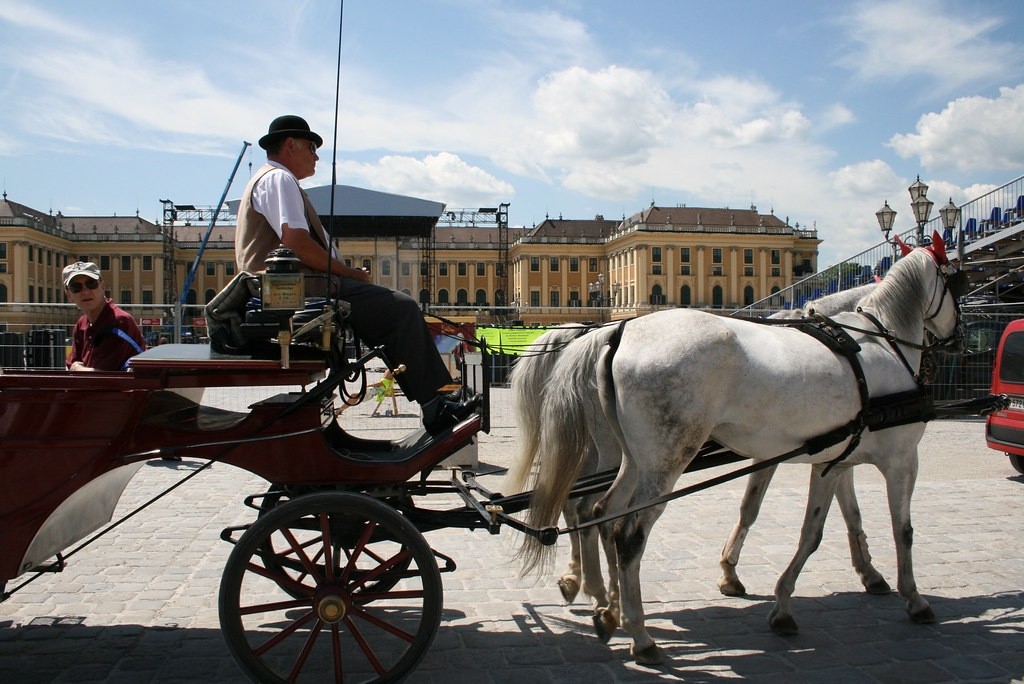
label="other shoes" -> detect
[162,456,182,461]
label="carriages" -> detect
[0,228,973,684]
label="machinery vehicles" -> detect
[145,140,253,348]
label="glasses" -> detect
[66,278,102,293]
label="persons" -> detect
[235,115,483,436]
[62,262,146,372]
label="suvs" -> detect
[983,317,1024,477]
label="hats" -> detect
[62,261,102,286]
[258,115,323,150]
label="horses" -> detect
[510,230,965,667]
[502,280,895,605]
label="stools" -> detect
[241,266,350,334]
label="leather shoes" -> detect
[422,392,479,436]
[443,389,462,402]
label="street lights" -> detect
[587,271,621,324]
